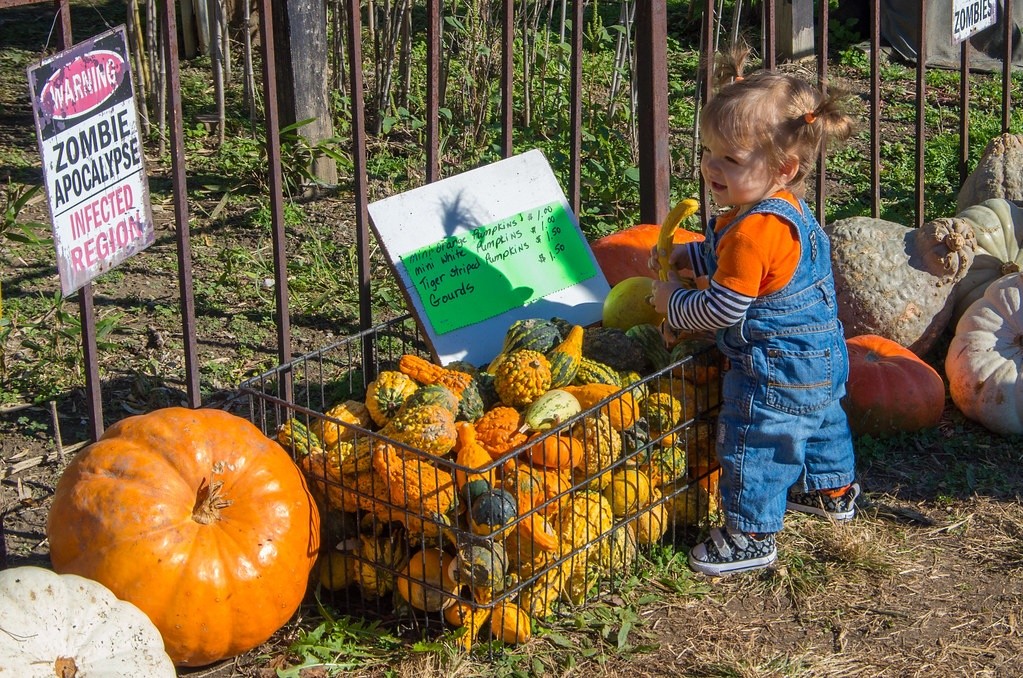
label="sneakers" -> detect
[787,482,860,520]
[688,524,777,575]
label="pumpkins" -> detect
[272,131,1023,657]
[0,566,177,678]
[45,406,322,667]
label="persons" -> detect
[650,47,860,576]
